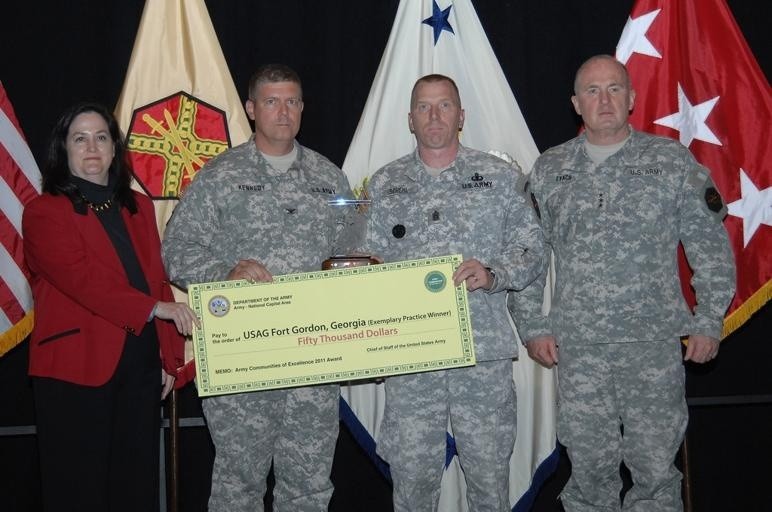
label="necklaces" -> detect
[85,198,111,213]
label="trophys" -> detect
[319,194,381,271]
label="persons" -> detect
[355,72,548,511]
[160,63,386,512]
[21,102,203,510]
[505,53,739,510]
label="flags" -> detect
[111,0,255,393]
[0,81,46,361]
[576,0,772,348]
[341,0,564,511]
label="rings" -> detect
[708,352,715,358]
[473,275,482,282]
